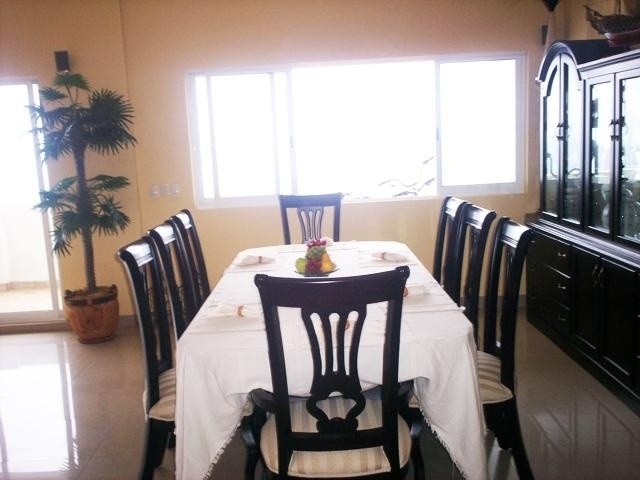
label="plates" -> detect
[293,265,341,277]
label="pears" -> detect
[320,252,331,272]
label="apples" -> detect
[295,258,306,272]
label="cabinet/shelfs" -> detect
[523,39,640,418]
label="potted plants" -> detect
[22,72,139,346]
